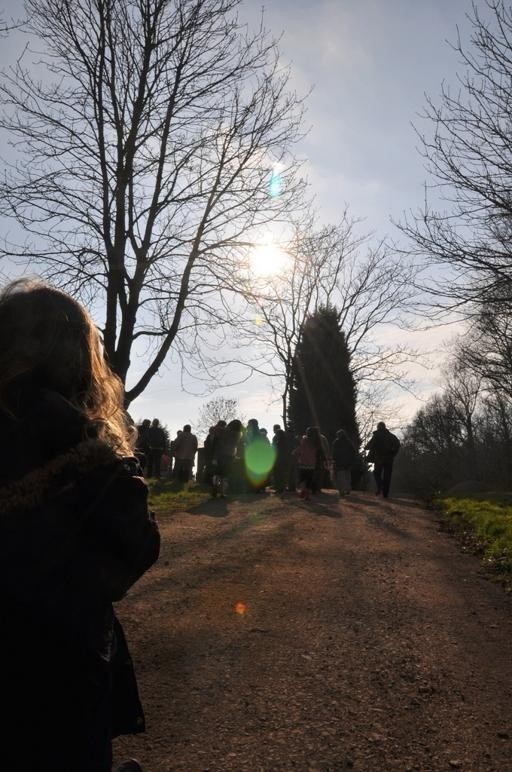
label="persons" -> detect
[0,276,160,771]
[125,417,401,501]
